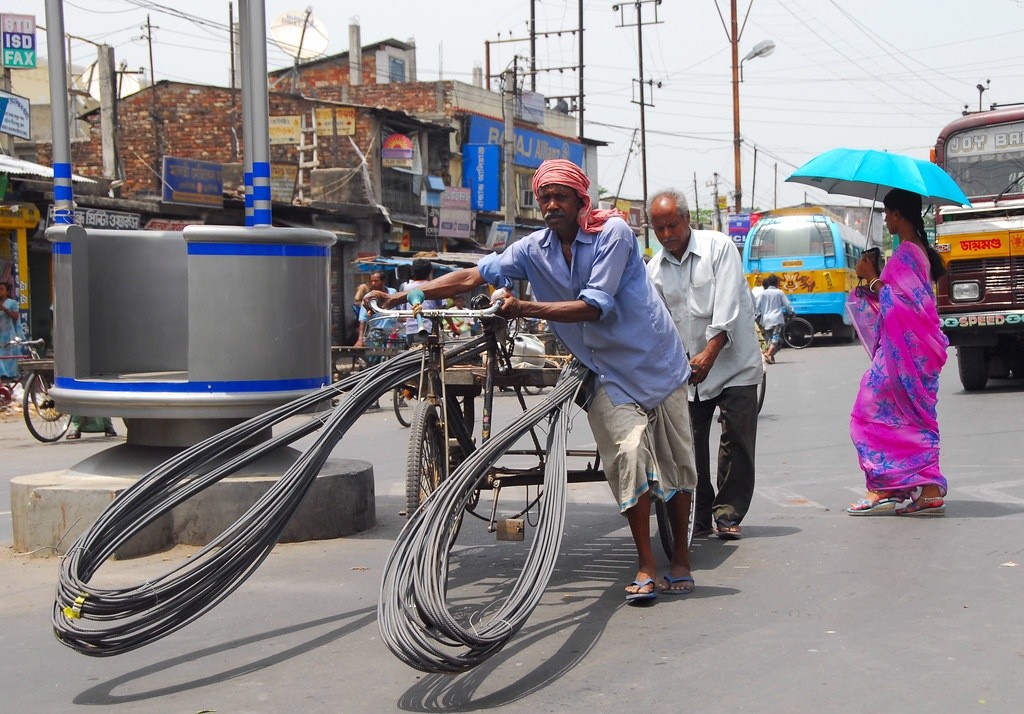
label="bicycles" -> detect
[756,313,815,354]
[393,332,464,427]
[0,337,72,442]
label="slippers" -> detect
[717,518,741,537]
[693,522,713,537]
[626,579,657,599]
[662,575,694,594]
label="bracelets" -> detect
[869,277,881,292]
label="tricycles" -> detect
[367,288,698,562]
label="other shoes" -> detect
[764,353,776,364]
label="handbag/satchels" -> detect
[855,247,880,297]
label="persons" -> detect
[0,281,25,394]
[751,275,796,364]
[847,189,949,516]
[644,190,764,539]
[362,158,699,599]
[354,258,474,400]
[66,415,117,439]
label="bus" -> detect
[743,206,872,344]
[930,106,1024,391]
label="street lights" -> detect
[733,42,777,262]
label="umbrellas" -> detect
[784,148,971,279]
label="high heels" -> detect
[847,498,897,516]
[898,496,945,516]
